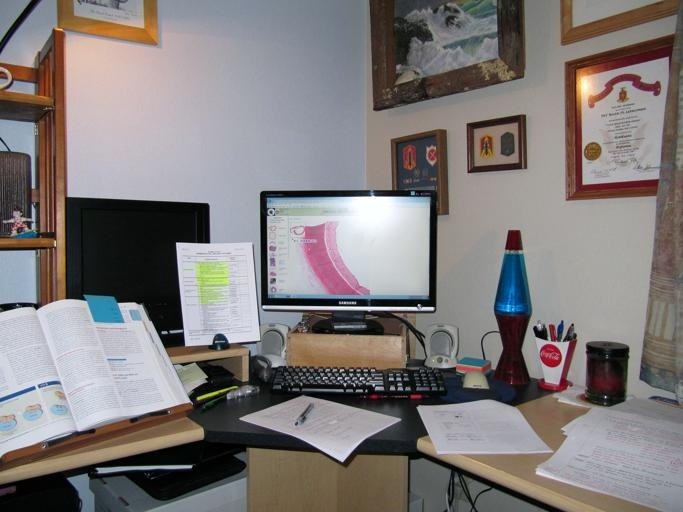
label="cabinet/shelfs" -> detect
[0,57,57,253]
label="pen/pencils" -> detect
[533,320,577,341]
[194,385,239,412]
[294,401,314,426]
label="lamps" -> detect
[479,227,535,390]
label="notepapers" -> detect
[456,357,493,376]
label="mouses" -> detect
[462,371,492,391]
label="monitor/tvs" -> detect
[259,189,440,335]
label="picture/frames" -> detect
[387,125,451,218]
[464,112,528,176]
[554,1,680,50]
[52,1,162,49]
[363,1,529,115]
[559,33,675,204]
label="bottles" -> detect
[582,340,630,406]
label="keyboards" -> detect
[272,365,446,398]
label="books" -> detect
[94,449,195,475]
[0,298,190,459]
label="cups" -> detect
[534,336,576,390]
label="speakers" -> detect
[424,323,460,369]
[257,322,288,367]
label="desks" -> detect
[1,314,681,510]
